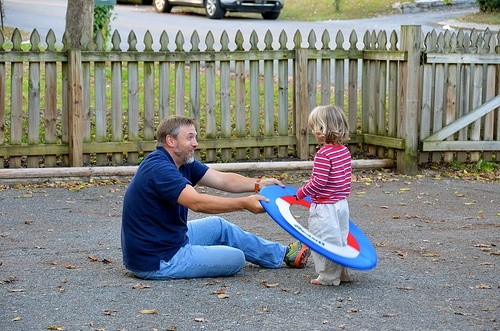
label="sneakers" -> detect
[284,239,310,267]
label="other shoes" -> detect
[340,267,353,283]
[310,278,322,286]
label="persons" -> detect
[120,115,313,280]
[295,105,355,286]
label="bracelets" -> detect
[254,175,263,193]
